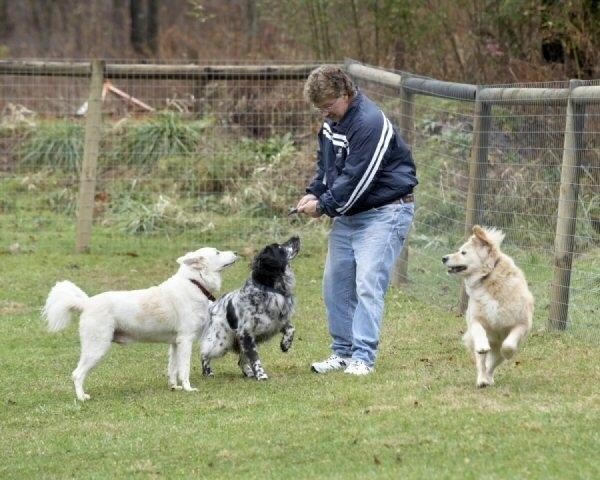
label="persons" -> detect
[295,63,418,376]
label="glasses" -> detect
[312,98,338,111]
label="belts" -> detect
[392,194,415,203]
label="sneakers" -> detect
[312,354,351,373]
[344,364,376,375]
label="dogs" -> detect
[440,224,536,389]
[199,233,302,382]
[39,245,238,404]
